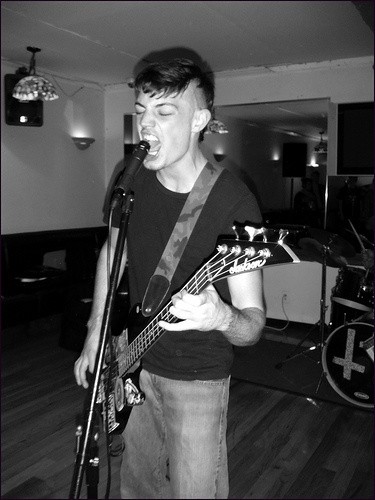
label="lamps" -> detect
[72,138,95,151]
[13,46,59,101]
[214,154,225,162]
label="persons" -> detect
[294,178,318,211]
[74,58,265,499]
[336,177,366,231]
[311,171,324,210]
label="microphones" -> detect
[112,141,151,207]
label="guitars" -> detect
[86,226,302,436]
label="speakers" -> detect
[282,142,307,178]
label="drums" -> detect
[330,264,375,311]
[321,318,374,408]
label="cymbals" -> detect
[308,227,357,259]
